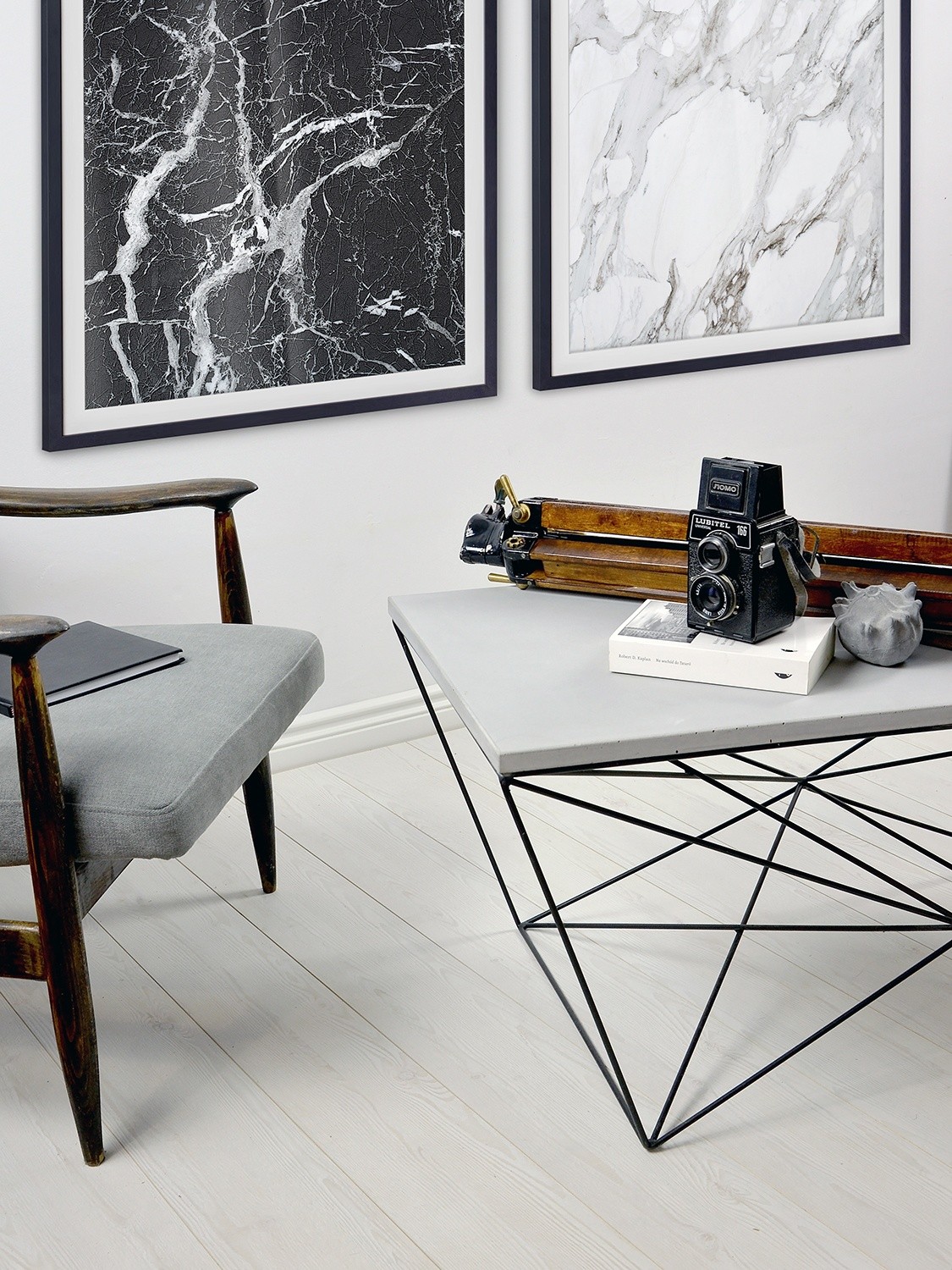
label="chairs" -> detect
[0,477,325,1167]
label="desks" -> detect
[388,587,952,1147]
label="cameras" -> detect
[685,457,800,646]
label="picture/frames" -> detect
[530,1,911,392]
[40,1,497,450]
